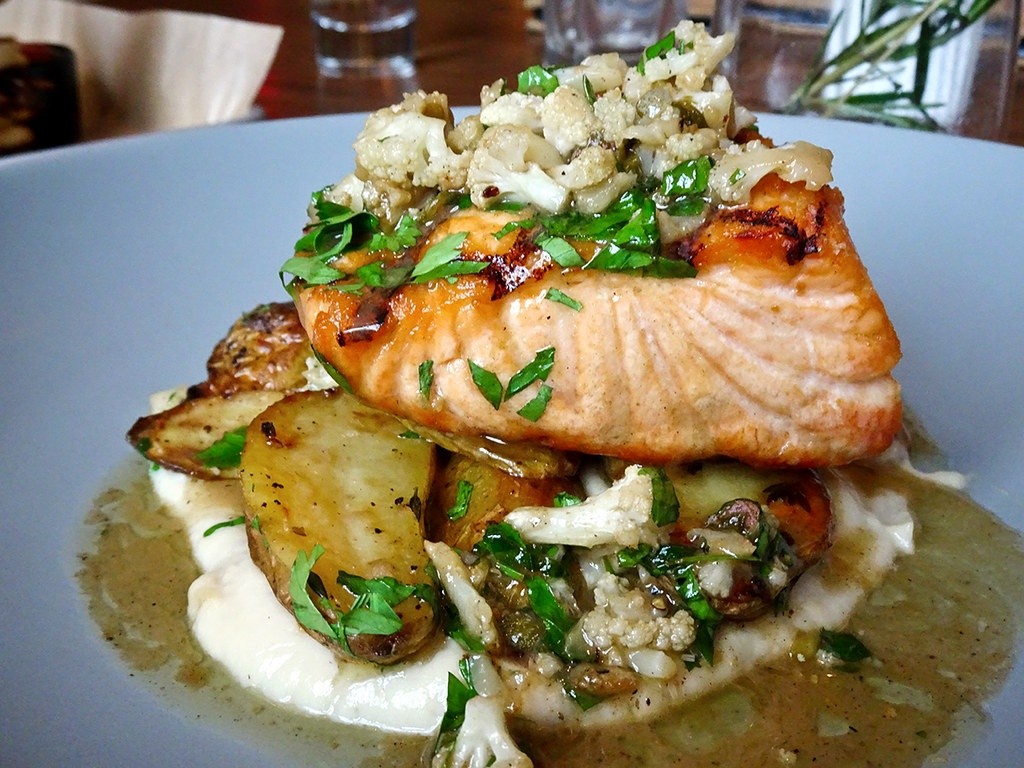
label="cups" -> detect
[308,0,417,80]
[712,1,836,116]
[538,1,674,75]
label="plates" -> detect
[0,106,1024,768]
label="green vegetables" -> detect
[134,31,873,758]
